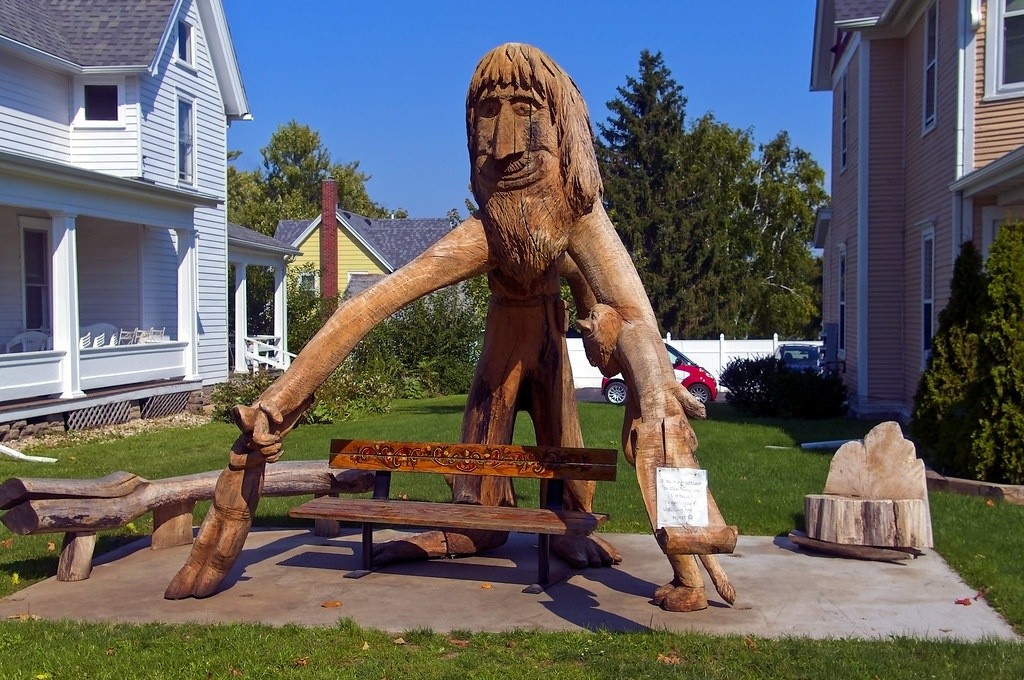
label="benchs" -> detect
[288,439,619,595]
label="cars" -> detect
[601,342,719,406]
[775,344,824,376]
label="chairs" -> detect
[6,322,165,354]
